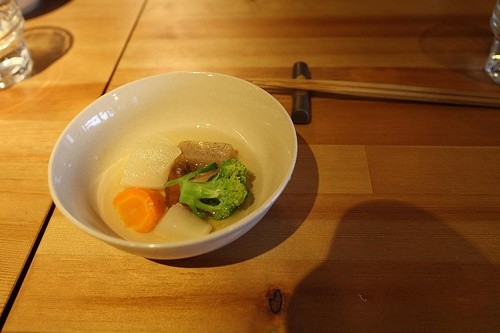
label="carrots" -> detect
[113,188,165,232]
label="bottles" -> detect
[484,1,500,85]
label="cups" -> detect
[0,0,33,90]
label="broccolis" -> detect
[164,159,249,219]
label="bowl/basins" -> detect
[48,71,297,260]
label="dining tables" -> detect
[0,0,500,333]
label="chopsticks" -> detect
[239,78,500,108]
